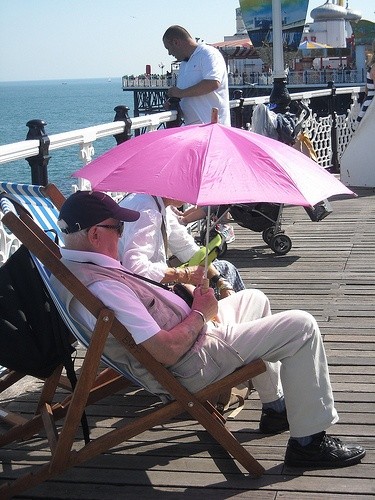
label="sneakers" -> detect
[176,215,187,227]
[171,205,182,216]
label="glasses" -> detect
[96,221,124,235]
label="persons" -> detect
[50,190,366,466]
[357,39,375,122]
[172,206,217,226]
[118,193,245,301]
[163,25,236,244]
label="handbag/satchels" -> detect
[173,283,193,309]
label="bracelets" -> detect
[211,273,225,283]
[218,282,233,294]
[184,268,190,284]
[173,267,179,283]
[192,310,206,326]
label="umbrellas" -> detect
[298,38,333,49]
[70,107,359,295]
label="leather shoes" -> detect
[260,407,289,433]
[284,432,366,467]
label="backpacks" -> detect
[0,245,76,380]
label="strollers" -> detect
[194,100,310,259]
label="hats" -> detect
[58,190,140,234]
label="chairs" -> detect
[0,191,267,496]
[1,182,122,436]
